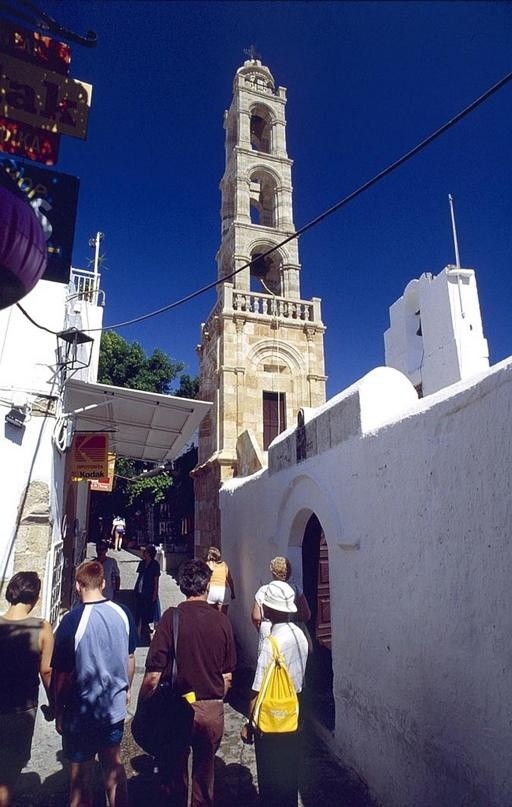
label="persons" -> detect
[92,540,120,601]
[250,555,311,632]
[0,572,56,807]
[241,580,310,807]
[134,545,161,645]
[202,545,236,615]
[49,560,136,806]
[110,511,128,551]
[137,560,237,806]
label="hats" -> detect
[258,580,298,614]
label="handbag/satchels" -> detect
[251,636,300,733]
[132,681,195,759]
